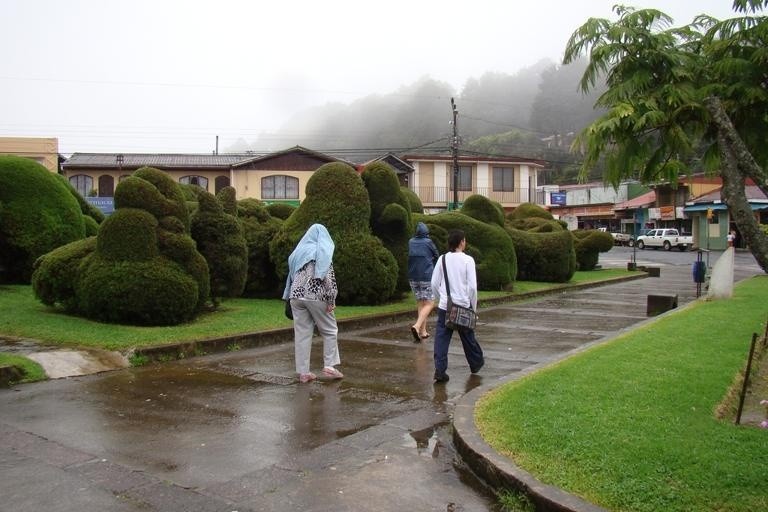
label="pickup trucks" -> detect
[628,228,695,251]
[597,227,631,246]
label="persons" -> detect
[430,230,484,383]
[281,223,344,383]
[727,231,734,247]
[407,221,439,342]
[730,228,736,245]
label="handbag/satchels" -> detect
[444,301,478,334]
[285,299,294,320]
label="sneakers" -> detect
[321,366,345,380]
[433,369,450,382]
[299,372,317,383]
[471,358,484,373]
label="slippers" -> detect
[411,325,431,341]
